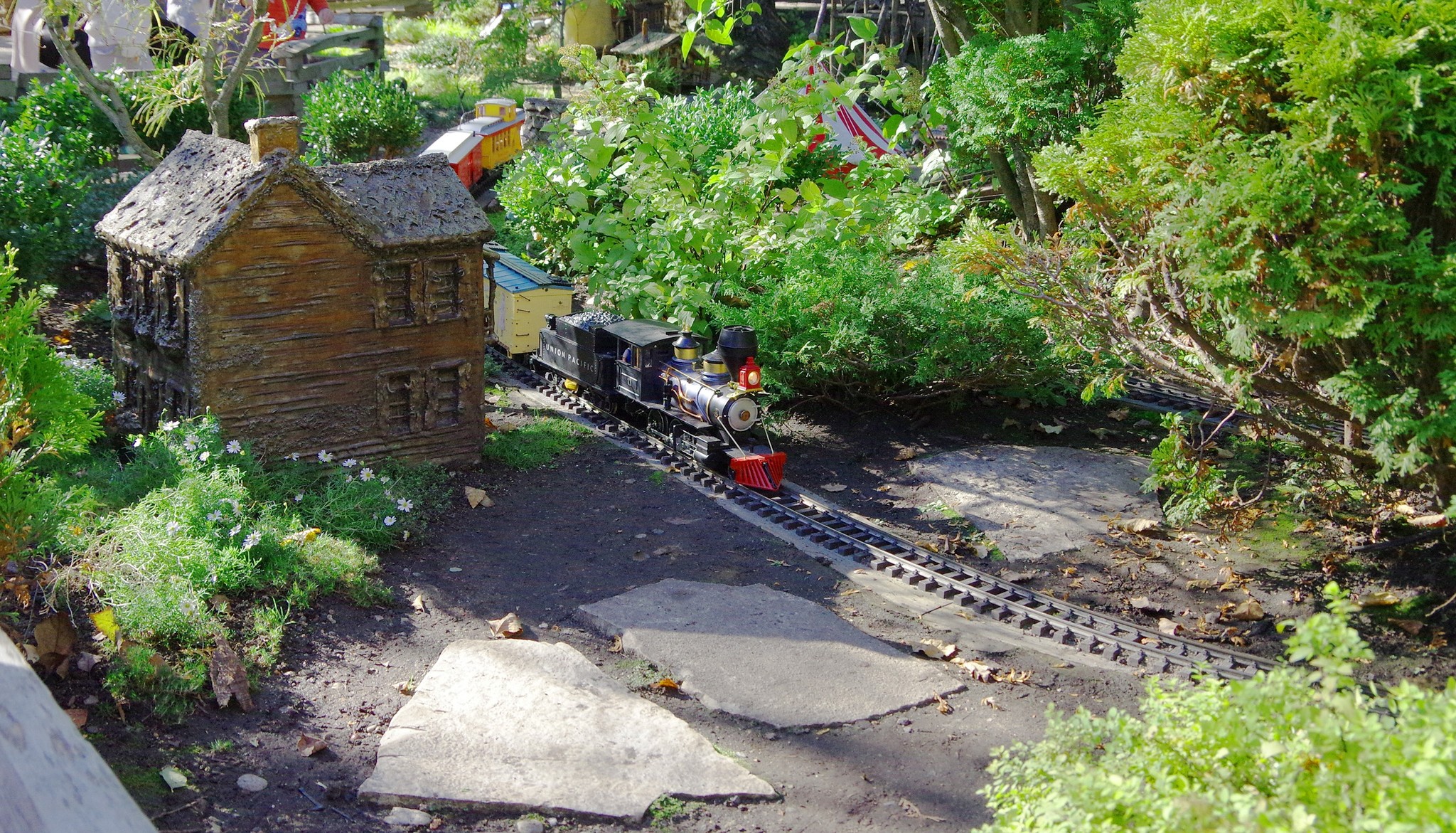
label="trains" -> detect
[419,98,788,491]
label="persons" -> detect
[237,0,334,69]
